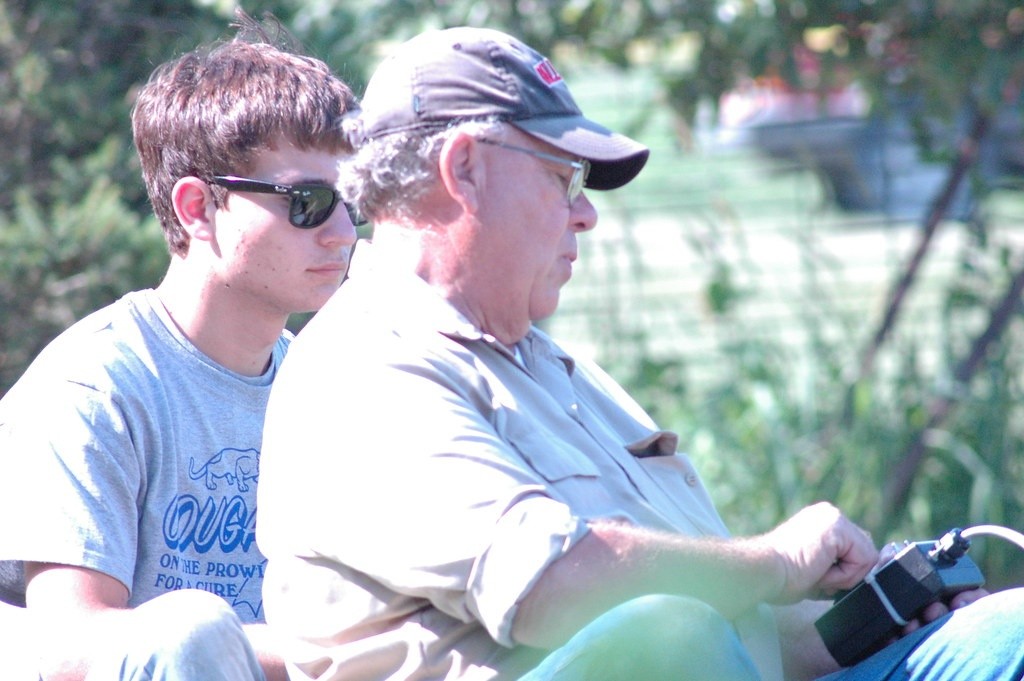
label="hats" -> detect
[349,27,650,192]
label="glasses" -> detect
[474,137,592,203]
[211,173,369,228]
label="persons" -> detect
[252,25,1022,681]
[0,34,378,681]
